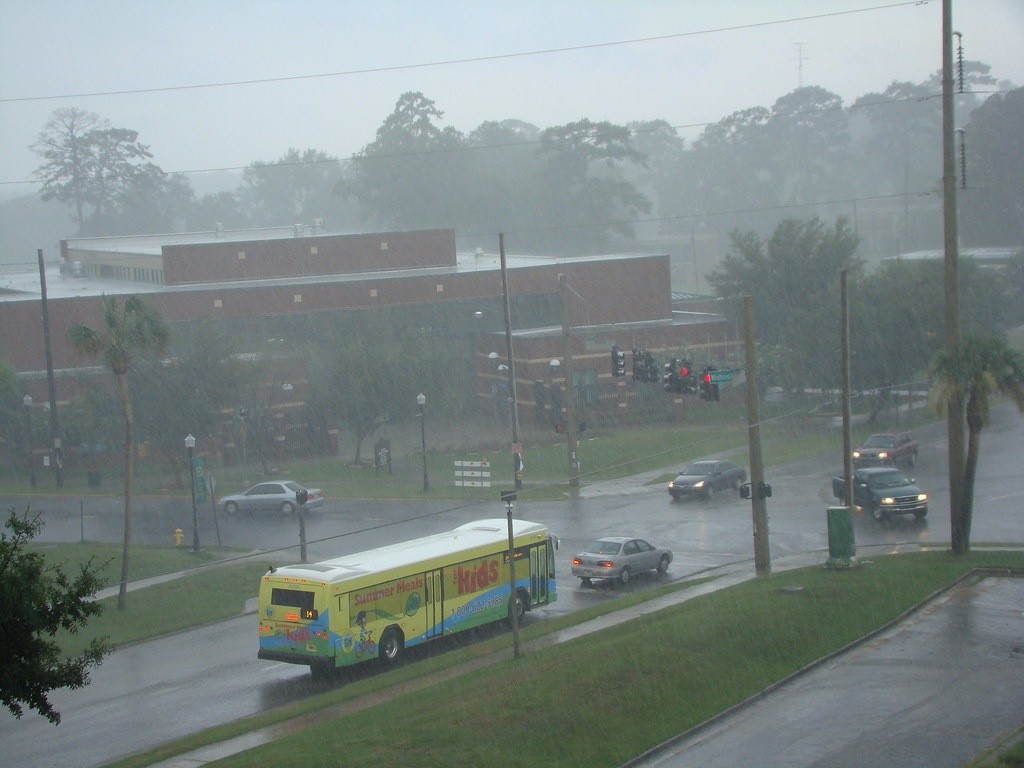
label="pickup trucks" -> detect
[832,468,929,521]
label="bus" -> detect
[255,515,560,673]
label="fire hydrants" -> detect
[172,529,183,546]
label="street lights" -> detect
[500,490,523,658]
[185,434,201,554]
[487,352,521,490]
[23,393,38,483]
[418,392,429,493]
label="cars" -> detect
[215,476,322,517]
[668,461,745,502]
[571,535,675,585]
[855,430,919,468]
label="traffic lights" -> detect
[633,351,659,384]
[611,348,623,375]
[556,423,565,432]
[663,360,678,392]
[678,360,720,402]
